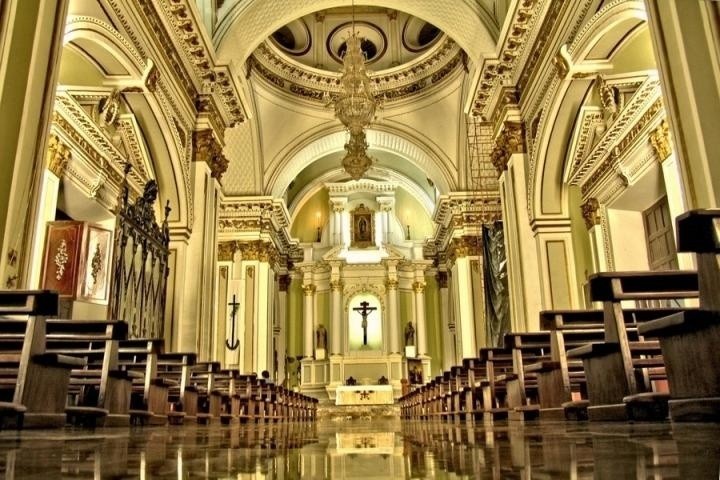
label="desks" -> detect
[336,386,393,405]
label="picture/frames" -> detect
[39,221,81,301]
[76,222,112,305]
[349,204,377,248]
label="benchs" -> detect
[0,289,87,429]
[496,329,637,421]
[156,362,222,424]
[451,366,585,421]
[524,308,699,423]
[567,271,699,424]
[69,352,198,426]
[474,347,552,421]
[459,357,551,421]
[190,370,241,426]
[638,209,720,422]
[0,338,178,426]
[0,319,144,427]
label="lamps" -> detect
[332,1,377,181]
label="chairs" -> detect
[424,385,428,419]
[266,383,276,424]
[429,380,435,420]
[314,398,318,420]
[257,379,267,424]
[307,397,311,422]
[275,386,283,422]
[378,376,388,385]
[294,393,299,422]
[299,394,303,421]
[439,376,444,420]
[311,397,315,420]
[346,376,356,385]
[283,388,288,422]
[288,390,293,422]
[398,388,420,420]
[434,378,438,419]
[303,395,306,422]
[420,388,423,419]
[443,371,452,420]
[234,375,259,423]
